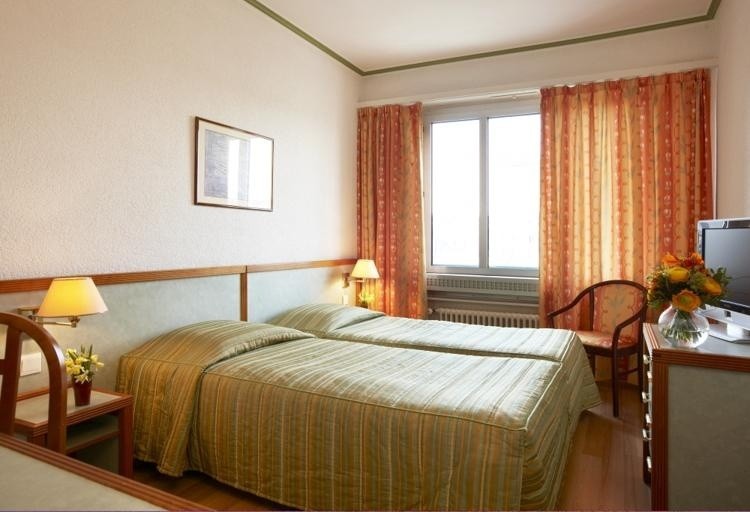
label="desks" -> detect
[0,429,222,512]
[640,324,749,507]
[15,380,134,476]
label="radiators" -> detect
[436,302,543,330]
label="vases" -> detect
[361,302,370,308]
[75,375,95,409]
[654,302,710,348]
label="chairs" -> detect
[547,280,644,413]
[0,311,68,453]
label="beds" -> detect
[118,321,573,512]
[266,299,603,432]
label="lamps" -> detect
[32,277,110,330]
[349,258,380,286]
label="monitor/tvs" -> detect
[698,219,750,344]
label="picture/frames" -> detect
[196,117,275,215]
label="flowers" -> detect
[646,245,728,310]
[359,288,379,300]
[70,348,102,382]
